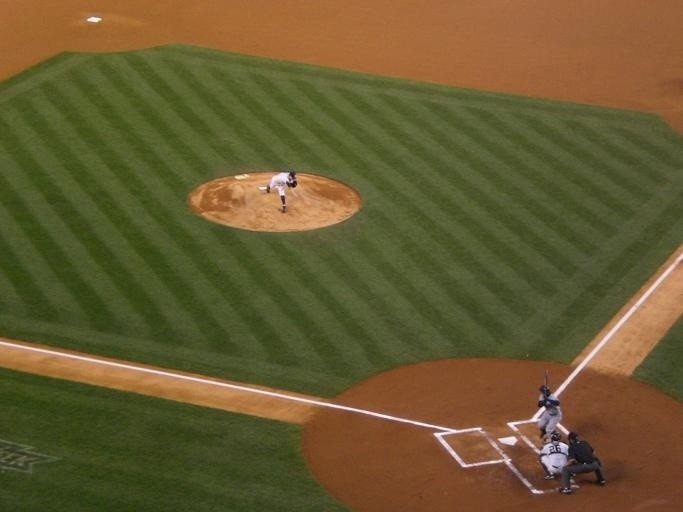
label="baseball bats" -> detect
[544,369,547,404]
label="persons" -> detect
[538,429,576,482]
[555,430,607,495]
[256,168,297,214]
[536,383,562,442]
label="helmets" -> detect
[550,430,561,441]
[539,384,549,392]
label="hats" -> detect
[567,431,577,438]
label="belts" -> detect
[551,465,559,468]
[582,462,593,465]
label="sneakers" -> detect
[543,473,606,493]
